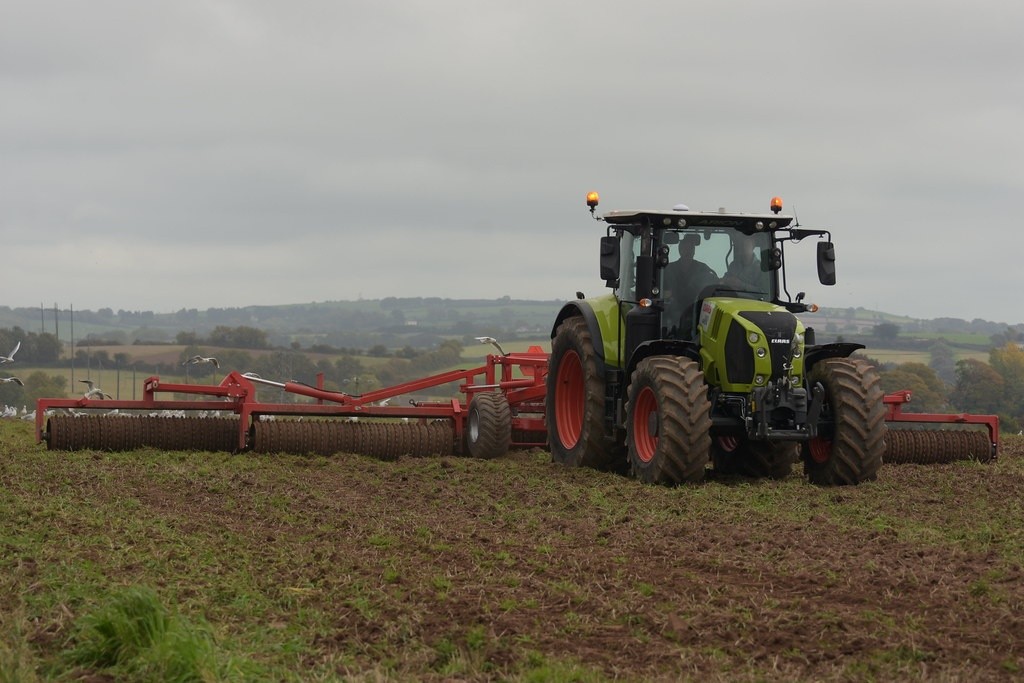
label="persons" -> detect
[727,236,760,281]
[666,238,712,291]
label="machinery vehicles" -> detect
[545,191,888,486]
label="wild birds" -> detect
[475,337,504,355]
[379,398,391,407]
[401,417,409,424]
[0,341,21,364]
[0,377,25,387]
[345,416,358,422]
[182,355,220,369]
[78,380,113,400]
[244,372,262,379]
[0,405,88,421]
[103,409,276,422]
